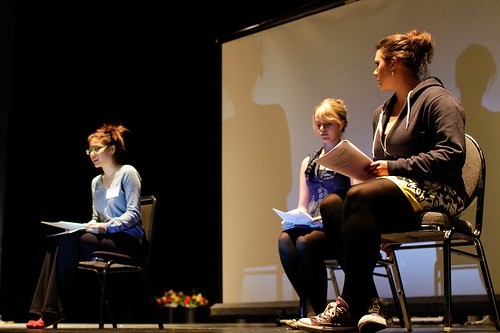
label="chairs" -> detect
[52,195,163,329]
[298,134,500,333]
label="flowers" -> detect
[154,288,209,310]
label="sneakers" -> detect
[357,297,395,333]
[296,295,356,333]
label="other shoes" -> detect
[285,319,299,330]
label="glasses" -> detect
[86,145,107,156]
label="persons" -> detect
[26,124,146,328]
[297,29,466,332]
[278,98,351,330]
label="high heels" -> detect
[27,319,58,329]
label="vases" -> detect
[160,305,207,324]
